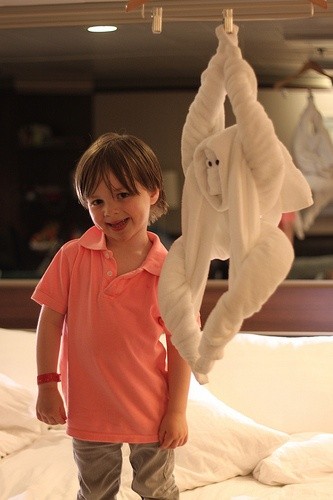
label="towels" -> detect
[290,94,333,239]
[157,24,314,385]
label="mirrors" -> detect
[0,0,333,280]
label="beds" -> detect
[0,327,333,500]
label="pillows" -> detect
[0,375,291,500]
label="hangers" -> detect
[273,61,333,93]
[124,0,328,34]
